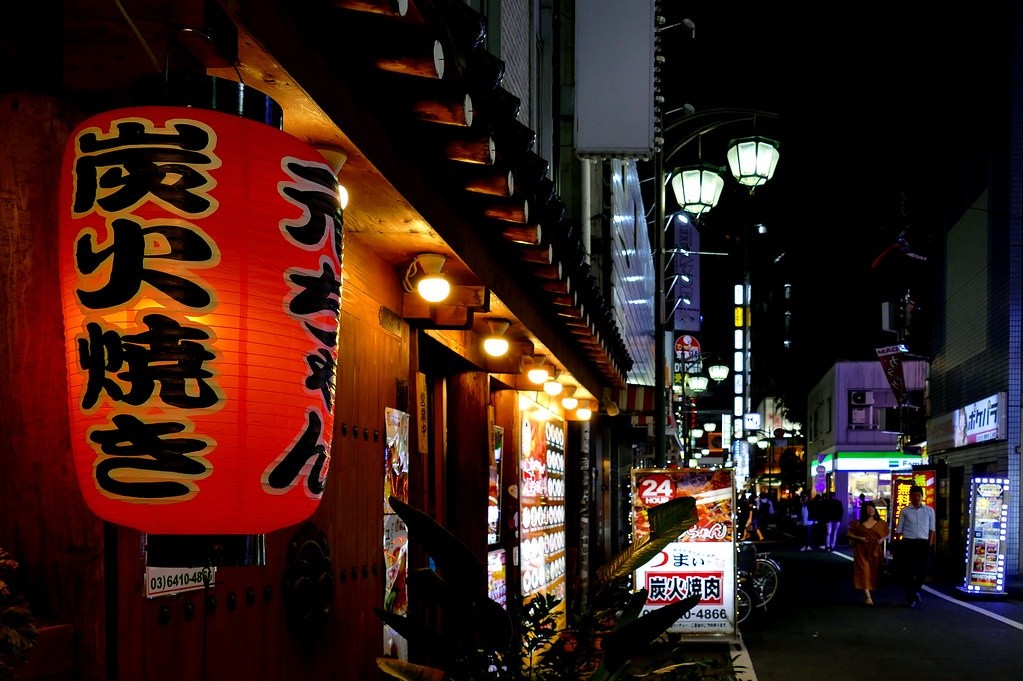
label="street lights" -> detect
[655,94,780,469]
[746,428,771,495]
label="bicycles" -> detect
[736,587,753,624]
[735,541,782,612]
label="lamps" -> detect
[480,318,513,355]
[313,148,348,212]
[528,353,550,385]
[414,253,451,311]
[542,370,561,396]
[576,400,592,420]
[603,396,620,417]
[561,386,578,409]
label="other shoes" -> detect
[807,546,812,550]
[914,592,922,603]
[800,546,806,551]
[909,599,916,608]
[864,596,873,605]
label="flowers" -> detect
[0,546,40,681]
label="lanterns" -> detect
[58,71,343,568]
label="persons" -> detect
[895,485,936,608]
[847,500,890,607]
[859,494,870,520]
[736,490,843,552]
